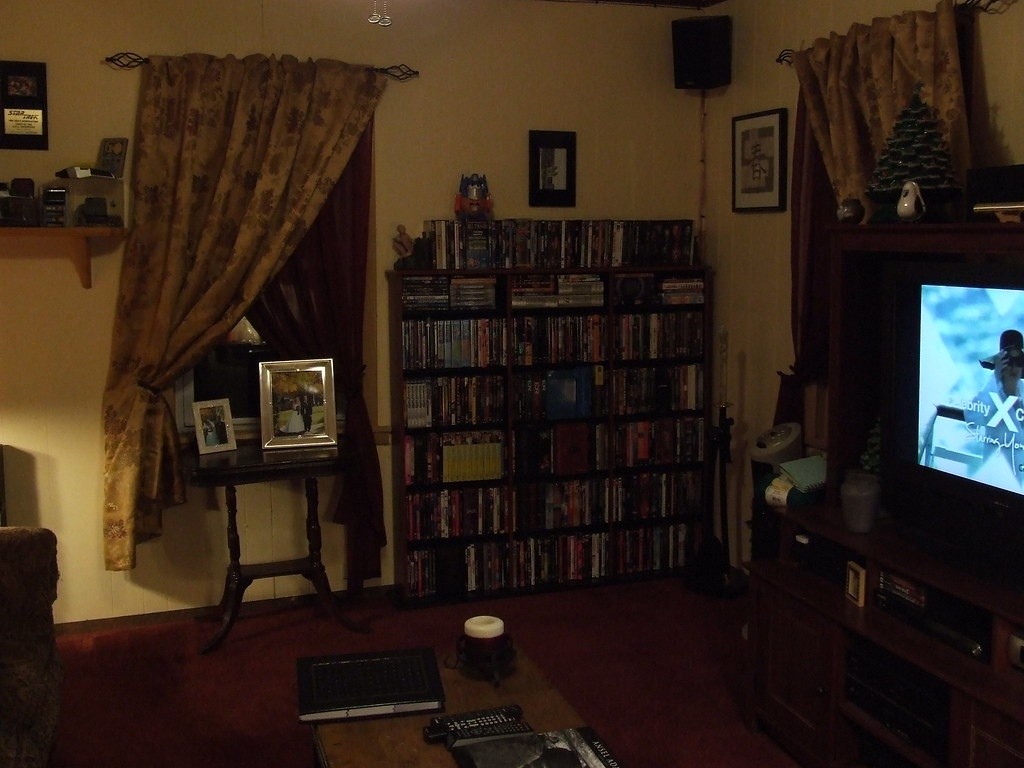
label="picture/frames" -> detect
[527,129,577,208]
[731,108,788,212]
[258,357,338,450]
[192,398,237,455]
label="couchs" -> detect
[0,525,70,768]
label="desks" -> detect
[177,433,371,657]
[309,646,586,768]
[826,219,1024,512]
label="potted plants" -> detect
[840,424,881,534]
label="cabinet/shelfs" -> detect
[741,506,1024,768]
[385,263,717,611]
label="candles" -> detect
[718,324,728,403]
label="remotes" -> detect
[422,704,534,752]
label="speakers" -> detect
[672,14,732,89]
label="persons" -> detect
[203,416,228,446]
[392,224,416,258]
[280,396,313,434]
[969,329,1024,482]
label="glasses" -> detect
[1003,355,1024,367]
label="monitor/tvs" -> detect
[890,273,1024,520]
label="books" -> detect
[296,647,447,725]
[402,219,706,598]
[779,452,828,492]
